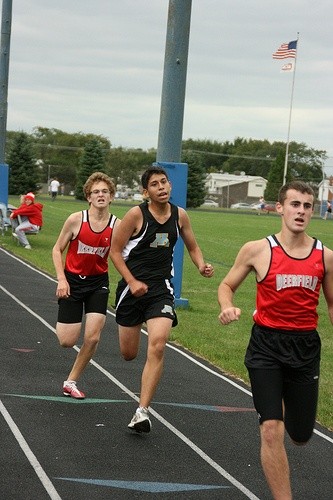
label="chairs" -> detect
[0,204,11,237]
[9,209,39,247]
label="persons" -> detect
[257,196,269,216]
[50,177,60,201]
[321,199,333,220]
[109,166,214,434]
[217,181,333,500]
[9,192,43,249]
[55,172,122,399]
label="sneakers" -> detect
[63,380,86,398]
[128,407,151,433]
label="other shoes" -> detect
[12,232,18,240]
[24,244,31,249]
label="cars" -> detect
[131,193,144,203]
[200,200,220,209]
[251,200,278,211]
[230,202,257,209]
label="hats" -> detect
[25,192,35,201]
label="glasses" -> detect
[90,188,111,195]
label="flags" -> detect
[273,40,296,59]
[281,61,296,73]
